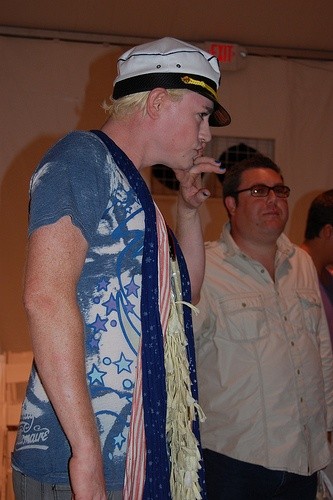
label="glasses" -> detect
[236,184,290,198]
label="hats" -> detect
[112,37,232,127]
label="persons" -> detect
[190,142,333,500]
[11,37,230,500]
[296,189,333,345]
